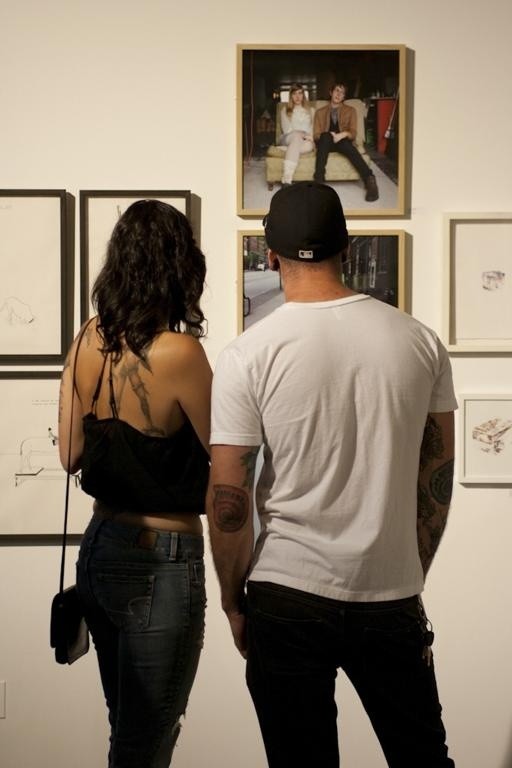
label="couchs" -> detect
[266,98,370,191]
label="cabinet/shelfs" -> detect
[368,96,397,154]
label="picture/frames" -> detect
[235,42,406,216]
[79,188,192,334]
[0,370,97,542]
[442,210,512,353]
[0,188,68,360]
[235,228,405,336]
[457,393,512,483]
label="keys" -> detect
[419,617,435,668]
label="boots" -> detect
[365,175,378,201]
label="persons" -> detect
[54,196,217,768]
[313,83,381,202]
[200,181,459,768]
[275,82,316,191]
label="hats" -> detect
[265,182,348,262]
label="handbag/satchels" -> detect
[50,586,90,665]
